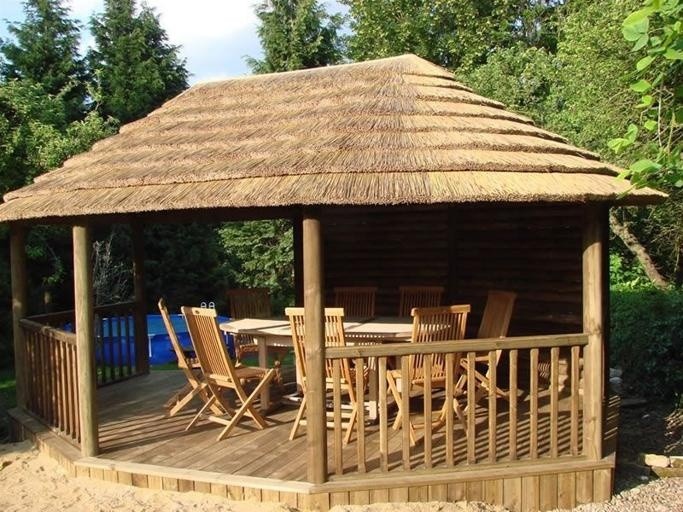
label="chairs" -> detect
[153,295,224,418]
[329,284,379,318]
[458,289,518,411]
[225,286,289,398]
[395,284,445,319]
[179,304,277,442]
[282,304,370,447]
[385,303,473,446]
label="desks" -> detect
[215,311,452,424]
